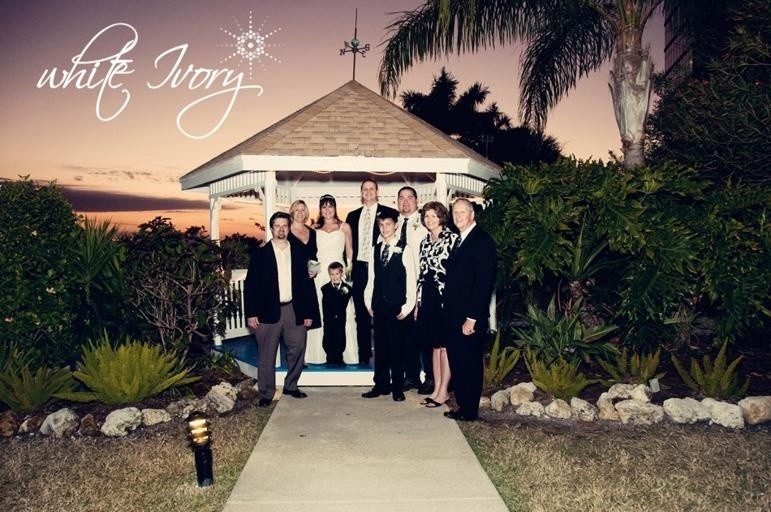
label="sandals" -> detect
[420,394,451,408]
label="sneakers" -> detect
[327,361,346,368]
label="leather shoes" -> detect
[392,388,405,402]
[443,410,477,422]
[259,397,271,407]
[361,388,391,398]
[283,388,307,399]
[403,377,435,394]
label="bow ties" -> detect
[333,283,339,285]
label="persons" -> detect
[342,179,399,368]
[282,199,321,367]
[397,186,436,396]
[319,262,354,369]
[242,210,313,407]
[308,193,360,367]
[360,211,419,402]
[411,201,461,409]
[441,197,497,423]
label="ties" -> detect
[381,244,390,265]
[362,208,372,261]
[455,236,462,250]
[400,218,409,244]
